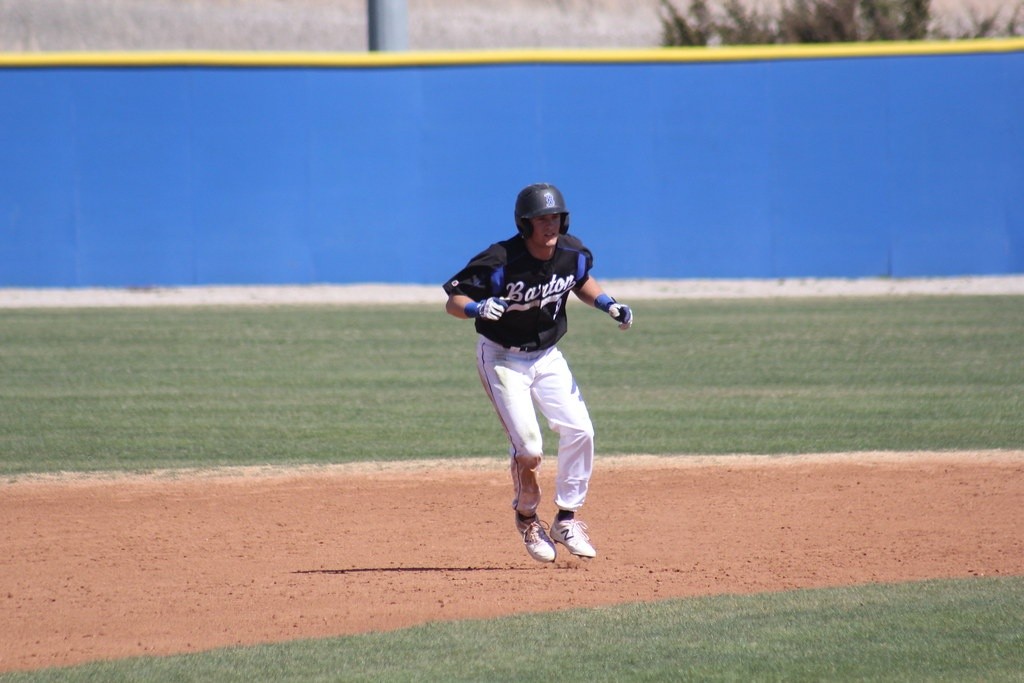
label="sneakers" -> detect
[549,514,596,557]
[514,511,557,562]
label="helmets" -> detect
[514,184,569,224]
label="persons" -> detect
[442,183,633,563]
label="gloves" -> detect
[606,296,633,330]
[477,297,510,321]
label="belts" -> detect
[503,345,539,352]
